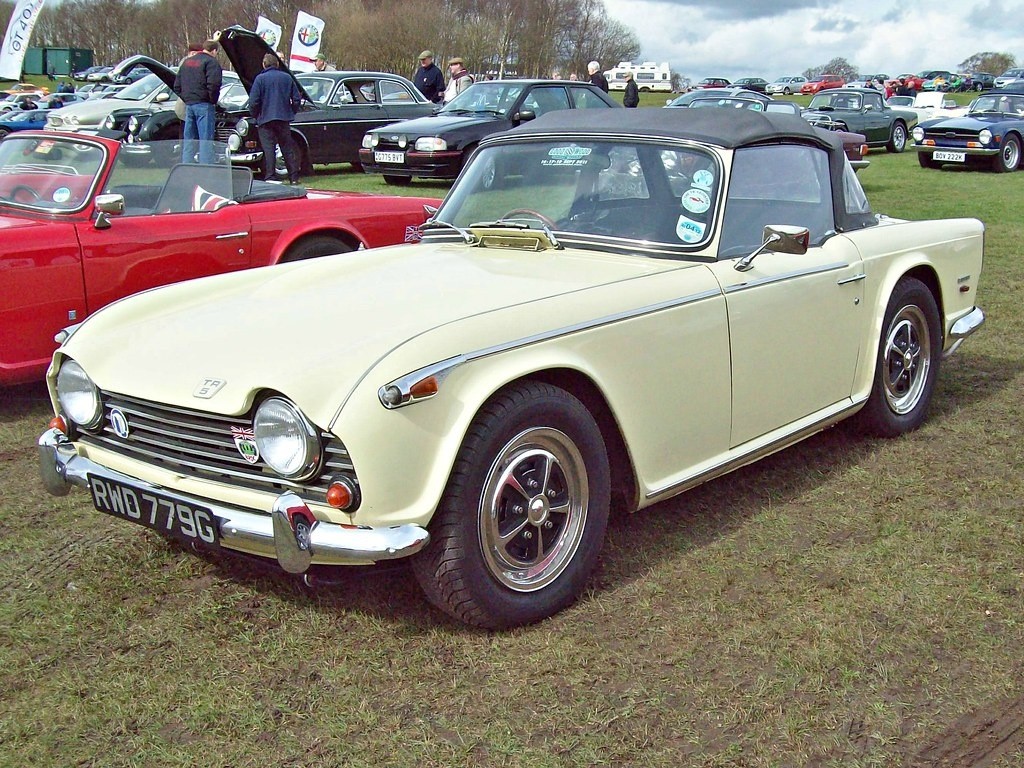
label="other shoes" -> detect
[291,180,301,186]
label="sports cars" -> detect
[685,97,871,173]
[0,129,443,393]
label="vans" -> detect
[602,69,673,93]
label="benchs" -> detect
[152,163,253,213]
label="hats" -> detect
[447,58,462,70]
[418,50,433,60]
[623,72,634,77]
[309,54,325,62]
[189,43,204,51]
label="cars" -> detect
[841,74,893,91]
[692,78,732,93]
[764,76,810,95]
[801,74,847,97]
[36,105,985,630]
[94,54,251,171]
[193,24,446,183]
[42,66,244,153]
[358,78,627,193]
[0,65,156,144]
[992,67,1024,90]
[909,89,1024,174]
[799,87,918,153]
[725,77,770,95]
[882,70,997,109]
[659,87,773,109]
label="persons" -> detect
[57,81,75,93]
[173,40,222,164]
[863,78,916,100]
[932,74,971,92]
[275,51,285,63]
[72,62,77,73]
[587,61,608,94]
[552,68,578,81]
[413,50,445,104]
[623,72,639,108]
[249,54,301,185]
[175,30,223,164]
[19,66,25,83]
[309,54,338,72]
[49,95,63,109]
[438,58,473,106]
[21,97,38,110]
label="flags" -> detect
[289,10,325,73]
[0,0,45,81]
[254,15,282,52]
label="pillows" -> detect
[192,183,239,211]
